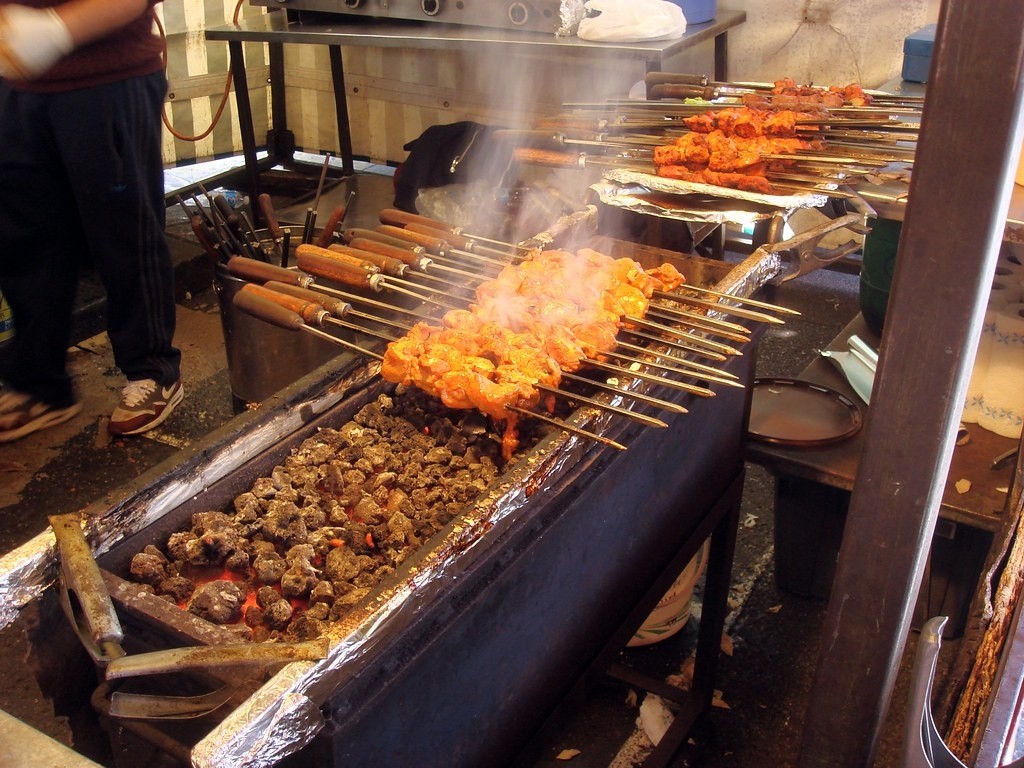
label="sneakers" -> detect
[0,386,81,442]
[107,368,182,435]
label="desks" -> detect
[204,9,747,243]
[741,311,1024,641]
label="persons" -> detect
[0,1,184,443]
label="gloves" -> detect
[0,3,75,80]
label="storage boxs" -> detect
[901,23,939,84]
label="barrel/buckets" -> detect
[209,225,355,405]
[627,536,709,646]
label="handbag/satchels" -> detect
[394,121,523,238]
